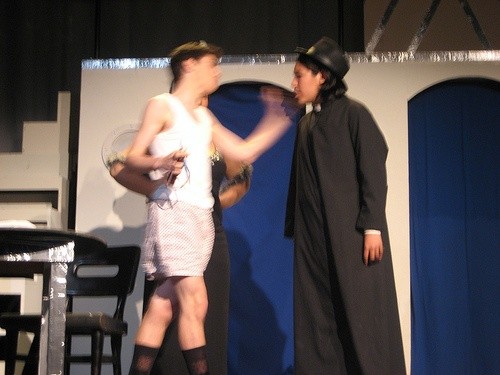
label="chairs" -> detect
[0,245,141,375]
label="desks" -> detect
[0,228,107,375]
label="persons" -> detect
[109,92,252,374]
[284,39,406,374]
[125,42,295,374]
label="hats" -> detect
[299,35,352,89]
